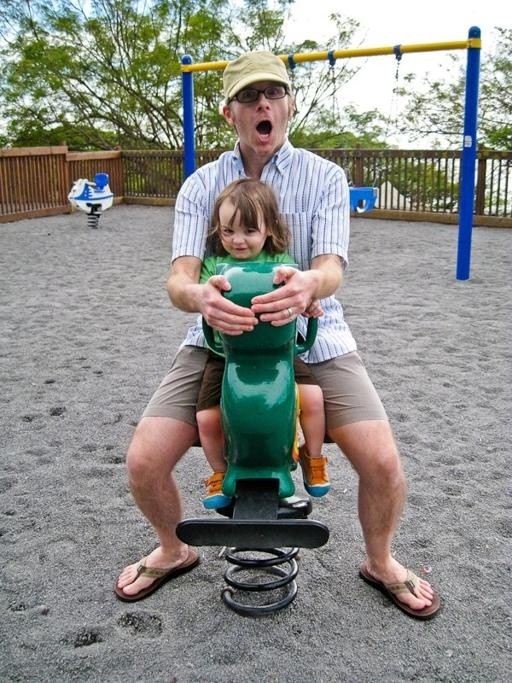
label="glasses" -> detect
[229,84,286,104]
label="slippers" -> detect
[112,543,201,603]
[359,560,441,621]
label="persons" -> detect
[107,48,443,622]
[191,176,336,509]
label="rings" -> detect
[287,308,293,316]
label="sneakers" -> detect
[299,443,331,498]
[202,471,233,510]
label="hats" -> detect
[223,49,291,104]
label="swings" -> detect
[326,45,402,213]
